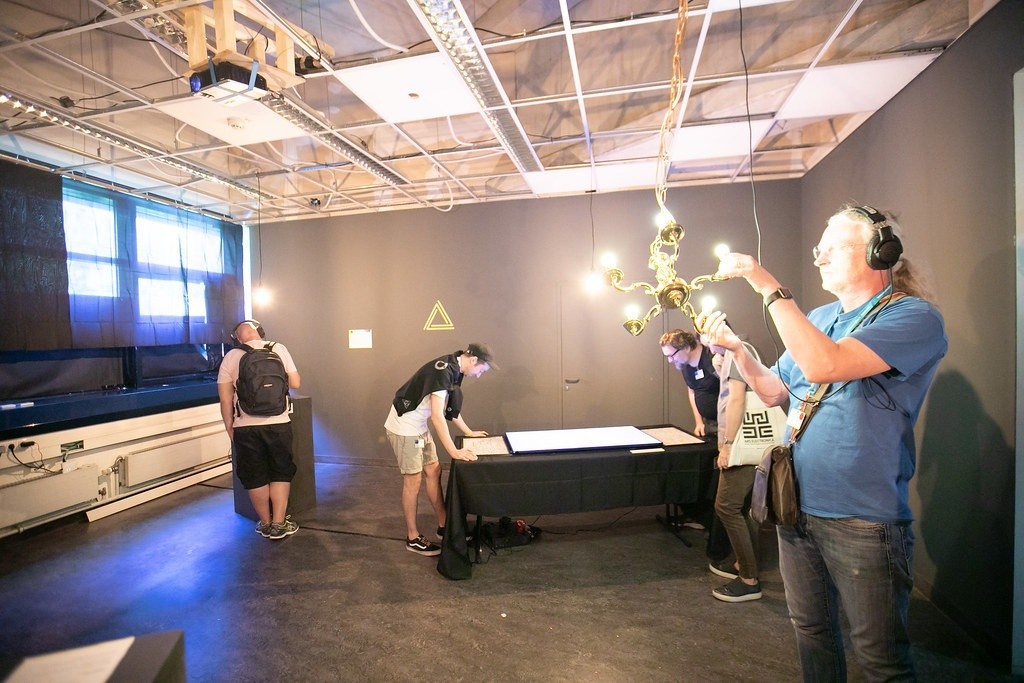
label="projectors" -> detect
[189,61,268,106]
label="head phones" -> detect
[231,319,265,347]
[852,205,906,274]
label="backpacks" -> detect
[233,341,289,416]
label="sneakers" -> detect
[270,515,299,539]
[255,519,272,537]
[712,577,762,602]
[671,513,705,530]
[704,529,711,540]
[436,525,473,541]
[709,559,740,580]
[406,534,441,556]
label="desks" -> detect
[437,425,732,582]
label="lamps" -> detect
[595,0,732,337]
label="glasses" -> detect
[813,244,869,260]
[664,348,681,360]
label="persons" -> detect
[696,318,762,602]
[383,343,500,556]
[659,329,724,539]
[216,320,300,539]
[695,202,948,683]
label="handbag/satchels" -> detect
[750,445,798,526]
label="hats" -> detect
[467,341,500,371]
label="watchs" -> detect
[763,287,793,307]
[723,437,734,445]
